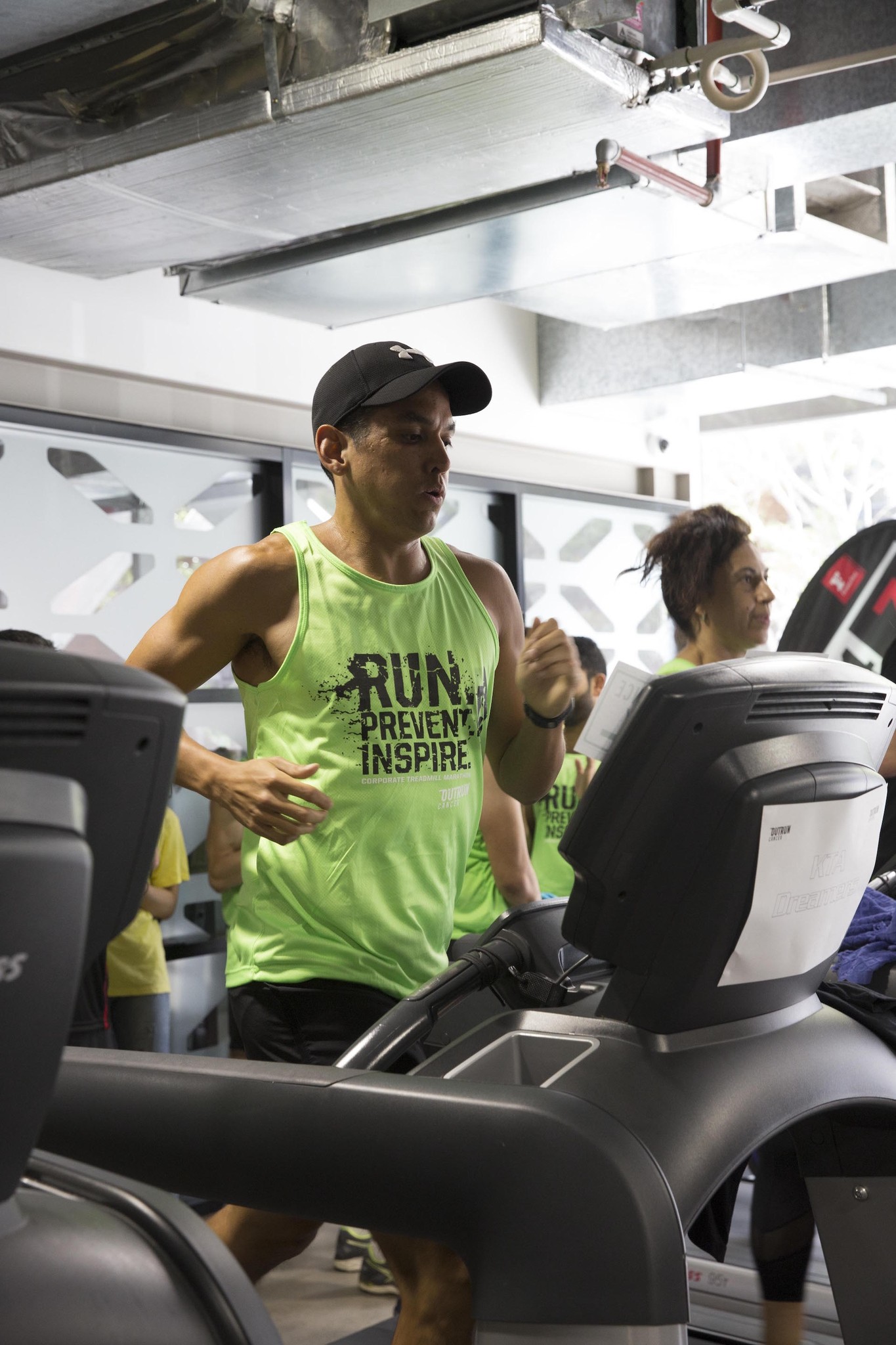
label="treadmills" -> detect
[0,639,896,1345]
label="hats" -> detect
[313,341,492,448]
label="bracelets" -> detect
[524,696,575,730]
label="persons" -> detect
[121,341,590,1344]
[447,635,609,939]
[67,795,242,1053]
[620,503,895,1345]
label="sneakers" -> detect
[359,1244,401,1294]
[333,1228,373,1271]
